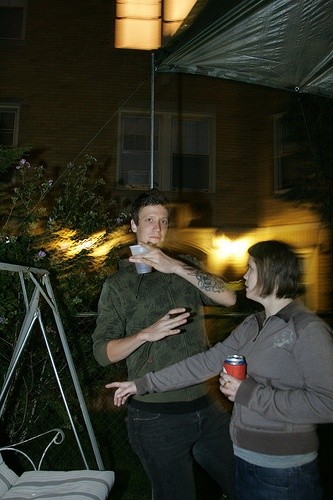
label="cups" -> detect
[129,245,152,275]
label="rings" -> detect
[222,380,230,386]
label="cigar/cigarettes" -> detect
[164,313,180,319]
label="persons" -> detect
[93,189,239,500]
[106,240,333,500]
